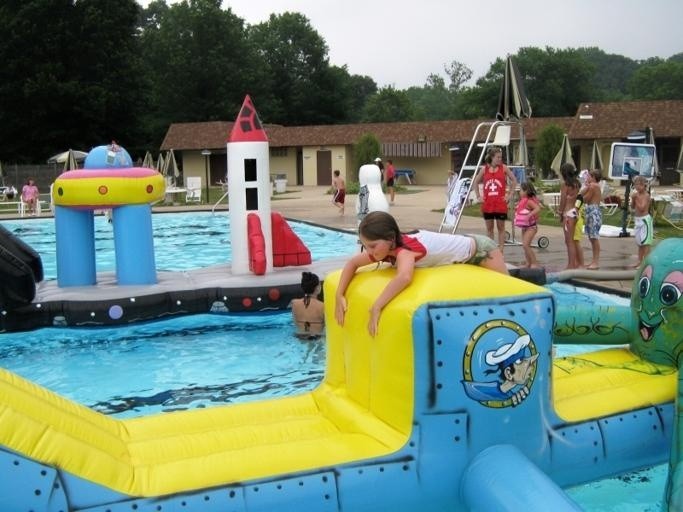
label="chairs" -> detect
[18,193,41,218]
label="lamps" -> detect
[202,149,212,203]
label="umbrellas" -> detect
[163,152,170,175]
[156,153,164,172]
[142,150,154,169]
[590,140,604,170]
[46,150,89,164]
[550,134,577,177]
[496,52,533,166]
[163,149,180,178]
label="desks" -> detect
[167,188,187,206]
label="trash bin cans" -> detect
[273,173,288,194]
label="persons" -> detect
[290,270,324,339]
[2,183,18,200]
[334,210,510,338]
[386,158,396,202]
[474,146,654,270]
[374,157,384,182]
[330,170,345,216]
[21,177,40,216]
